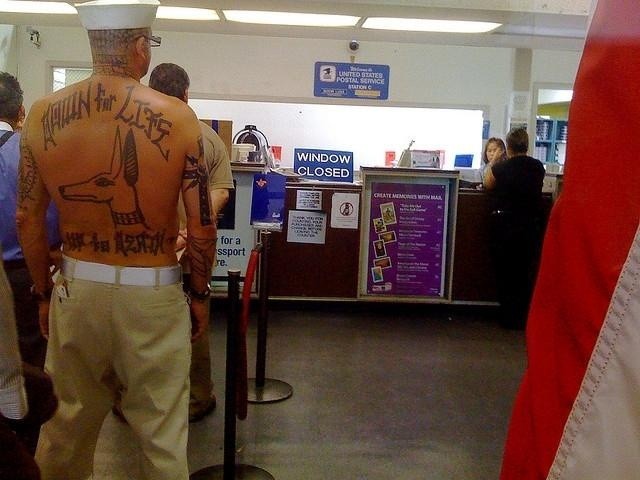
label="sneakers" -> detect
[189,392,217,423]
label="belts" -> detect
[61,254,181,289]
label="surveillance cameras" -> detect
[28,34,38,42]
[347,40,360,53]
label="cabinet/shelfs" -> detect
[536,118,568,166]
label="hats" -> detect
[74,1,160,31]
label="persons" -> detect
[498,1,640,480]
[114,63,235,422]
[481,128,548,305]
[1,71,61,480]
[19,0,219,479]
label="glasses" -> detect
[133,31,162,47]
